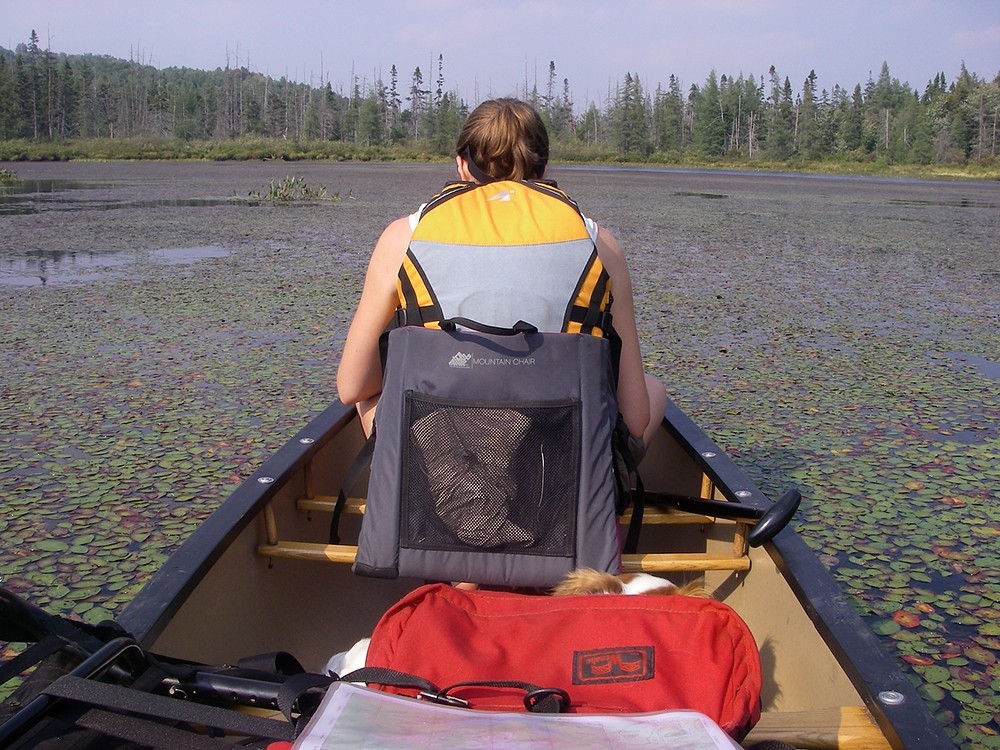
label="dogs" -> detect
[320,570,711,687]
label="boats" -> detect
[0,395,961,750]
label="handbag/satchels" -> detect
[364,583,761,744]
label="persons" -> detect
[337,98,665,519]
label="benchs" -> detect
[259,496,761,572]
[188,703,892,750]
